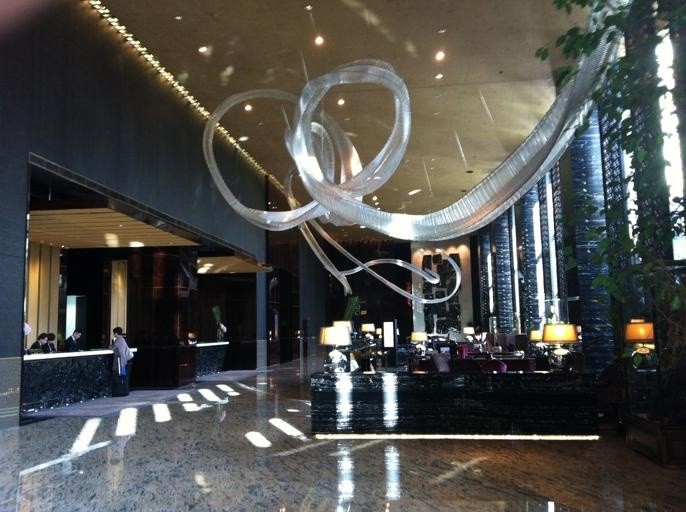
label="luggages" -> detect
[111,356,130,398]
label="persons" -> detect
[30,333,48,353]
[65,329,83,352]
[111,327,134,385]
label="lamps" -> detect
[376,327,382,335]
[626,321,655,343]
[332,320,353,333]
[362,323,375,333]
[320,326,352,346]
[412,331,428,342]
[530,329,543,342]
[541,323,579,346]
[463,326,475,335]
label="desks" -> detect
[429,351,536,374]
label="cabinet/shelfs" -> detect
[126,246,196,388]
[625,373,686,468]
